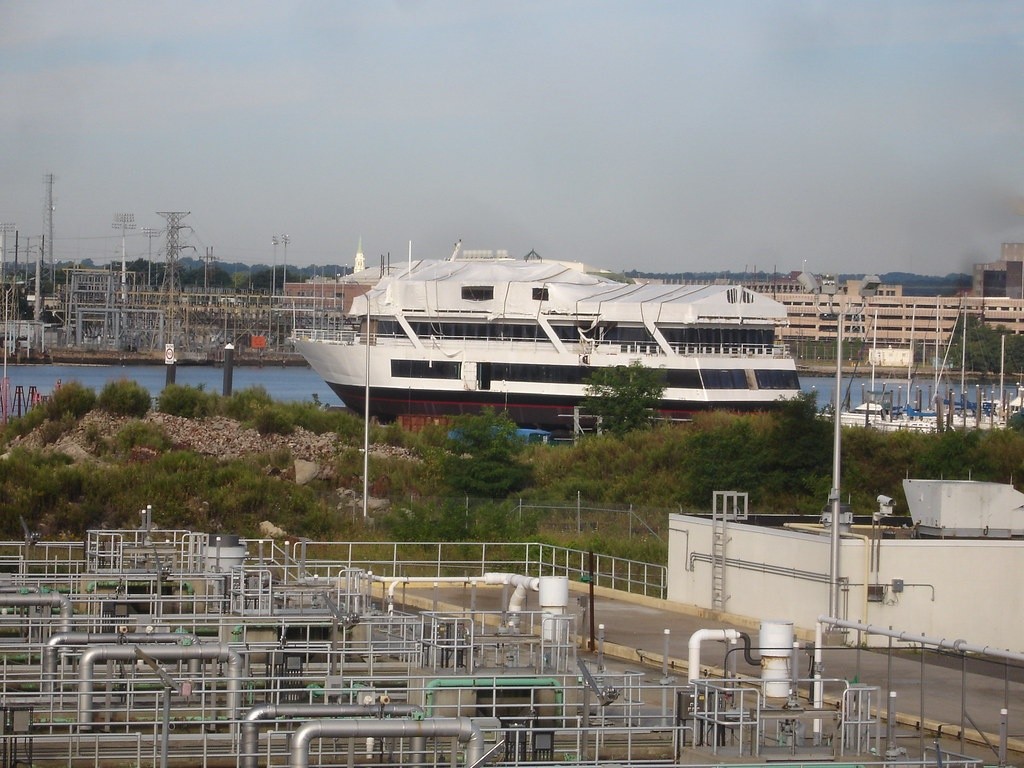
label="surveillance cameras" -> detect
[876,494,896,506]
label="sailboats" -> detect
[811,292,1024,434]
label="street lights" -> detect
[141,227,152,286]
[280,233,291,297]
[271,234,279,304]
[797,271,882,630]
[346,278,387,529]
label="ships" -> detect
[288,237,808,440]
